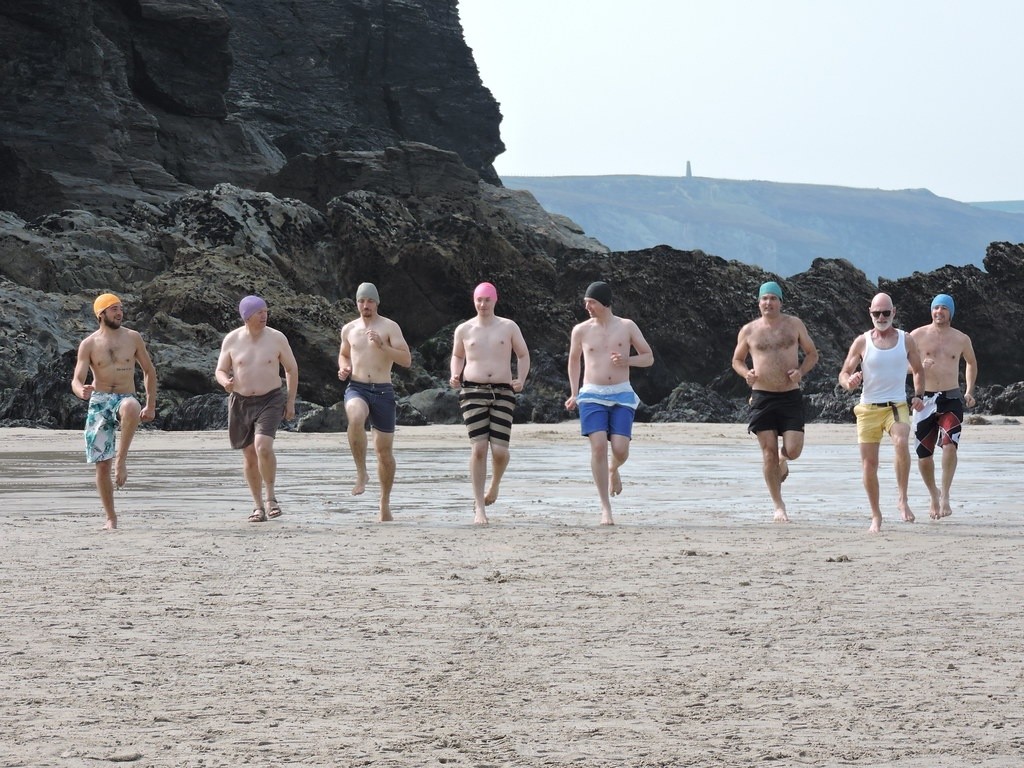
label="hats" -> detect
[759,282,783,303]
[930,294,954,320]
[585,281,613,308]
[238,295,266,320]
[93,293,121,319]
[356,282,380,305]
[473,282,497,304]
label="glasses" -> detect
[870,308,893,318]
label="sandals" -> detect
[265,498,281,518]
[248,508,267,522]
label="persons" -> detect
[215,296,297,521]
[72,295,157,528]
[732,282,819,520]
[565,282,654,526]
[907,295,977,520]
[838,292,925,533]
[450,282,529,523]
[338,282,411,522]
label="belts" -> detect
[872,402,899,421]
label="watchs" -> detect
[917,395,923,400]
